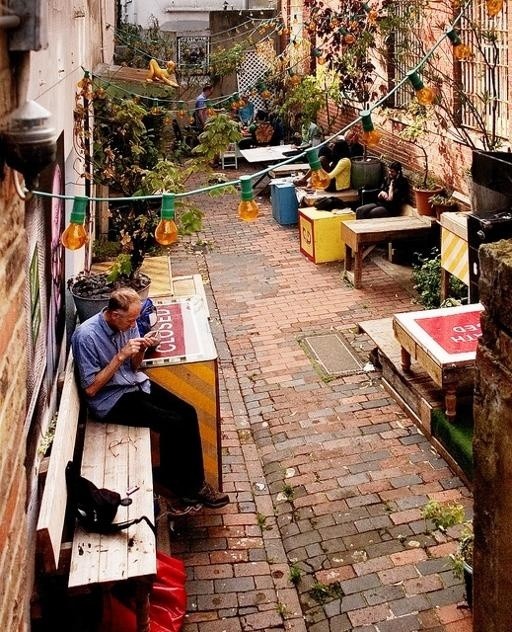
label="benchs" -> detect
[32,330,159,631]
[171,274,210,319]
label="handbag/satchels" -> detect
[65,461,132,533]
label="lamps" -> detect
[61,1,506,249]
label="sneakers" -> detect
[183,482,229,508]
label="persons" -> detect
[284,113,322,164]
[195,84,225,145]
[355,161,410,219]
[237,96,256,130]
[318,131,364,163]
[293,140,352,193]
[238,109,276,149]
[70,287,229,508]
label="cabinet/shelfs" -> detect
[439,210,475,305]
[297,206,357,265]
[133,293,224,493]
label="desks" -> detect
[388,300,490,419]
[88,256,173,300]
[338,214,439,290]
